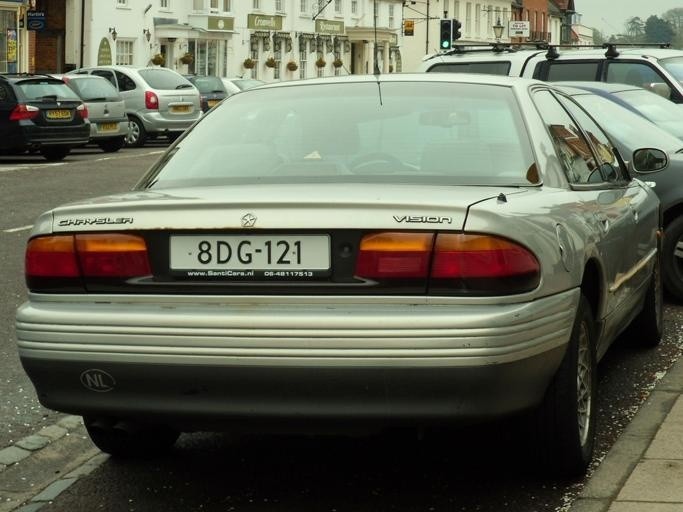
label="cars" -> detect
[183,73,265,113]
[361,40,683,303]
[11,72,672,484]
[46,73,129,154]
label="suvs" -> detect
[67,64,205,148]
[1,70,92,162]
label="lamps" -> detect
[109,27,118,41]
[144,29,152,41]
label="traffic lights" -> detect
[453,19,462,41]
[439,19,452,51]
